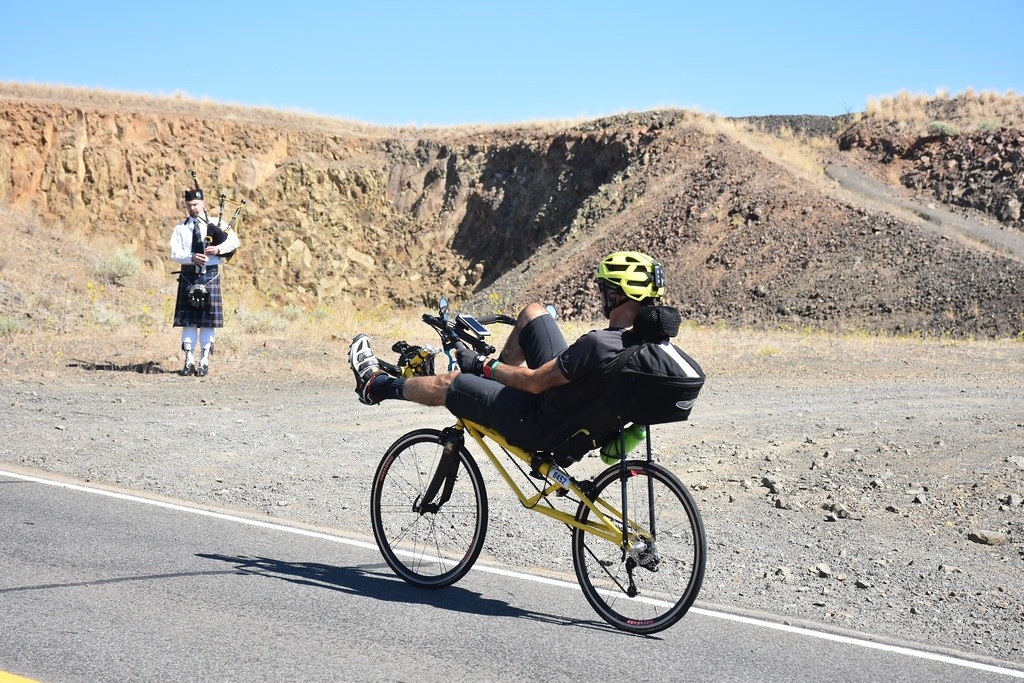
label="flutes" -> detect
[191,170,247,279]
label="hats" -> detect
[185,187,203,201]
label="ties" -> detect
[191,219,204,268]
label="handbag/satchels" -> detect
[180,271,219,308]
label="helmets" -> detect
[595,251,665,301]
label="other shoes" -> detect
[180,360,195,376]
[196,361,208,377]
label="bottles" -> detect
[599,426,645,465]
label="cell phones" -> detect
[458,313,492,336]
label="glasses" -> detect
[598,279,607,292]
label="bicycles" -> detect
[369,298,707,634]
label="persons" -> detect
[348,251,665,449]
[170,189,239,378]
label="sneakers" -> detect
[348,333,388,405]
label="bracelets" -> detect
[483,359,501,381]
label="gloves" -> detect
[454,349,488,377]
